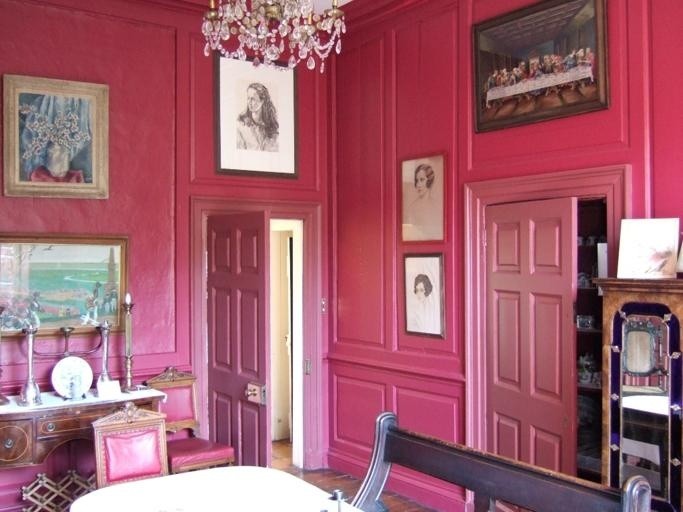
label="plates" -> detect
[50,355,93,400]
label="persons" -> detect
[483,47,596,107]
[407,272,435,325]
[236,81,280,150]
[402,163,443,210]
[1,279,119,332]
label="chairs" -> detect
[90,366,235,490]
[622,405,668,497]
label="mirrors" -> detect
[624,327,656,376]
[609,303,682,511]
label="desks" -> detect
[485,66,593,108]
[621,394,669,468]
[1,384,165,468]
[68,465,363,512]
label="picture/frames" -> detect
[1,232,129,339]
[212,49,298,179]
[471,1,610,133]
[0,73,111,200]
[399,151,449,245]
[402,252,445,340]
